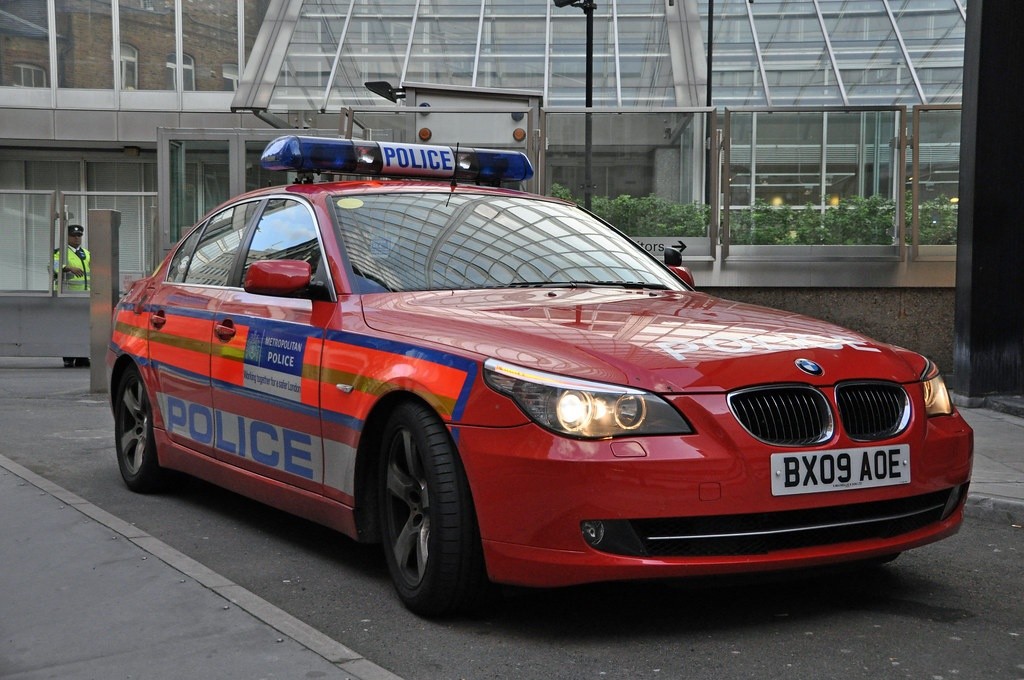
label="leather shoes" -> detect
[75,360,91,368]
[63,361,74,368]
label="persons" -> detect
[49,224,92,368]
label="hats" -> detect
[67,225,84,234]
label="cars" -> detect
[104,134,976,620]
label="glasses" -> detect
[69,234,82,237]
